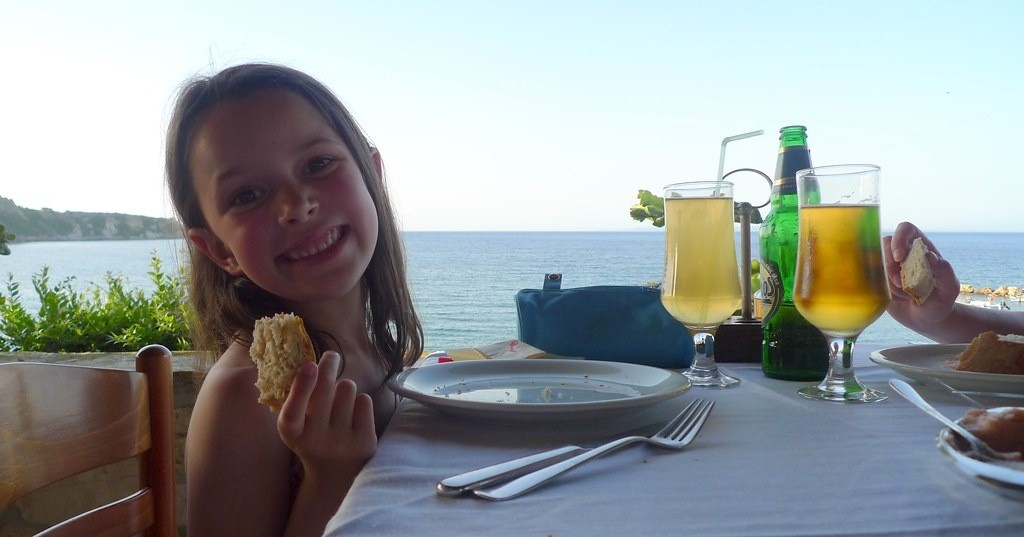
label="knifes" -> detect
[436,419,671,497]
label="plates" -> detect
[868,342,1024,393]
[384,359,692,424]
[936,406,1024,487]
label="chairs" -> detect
[0,344,177,537]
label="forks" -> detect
[888,378,1024,467]
[472,397,717,501]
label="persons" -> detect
[882,221,1024,345]
[163,65,423,537]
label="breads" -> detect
[956,332,1024,375]
[250,314,316,409]
[898,236,935,304]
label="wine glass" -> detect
[659,181,743,390]
[792,164,892,404]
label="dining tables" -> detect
[322,345,1024,537]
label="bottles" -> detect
[758,125,830,382]
